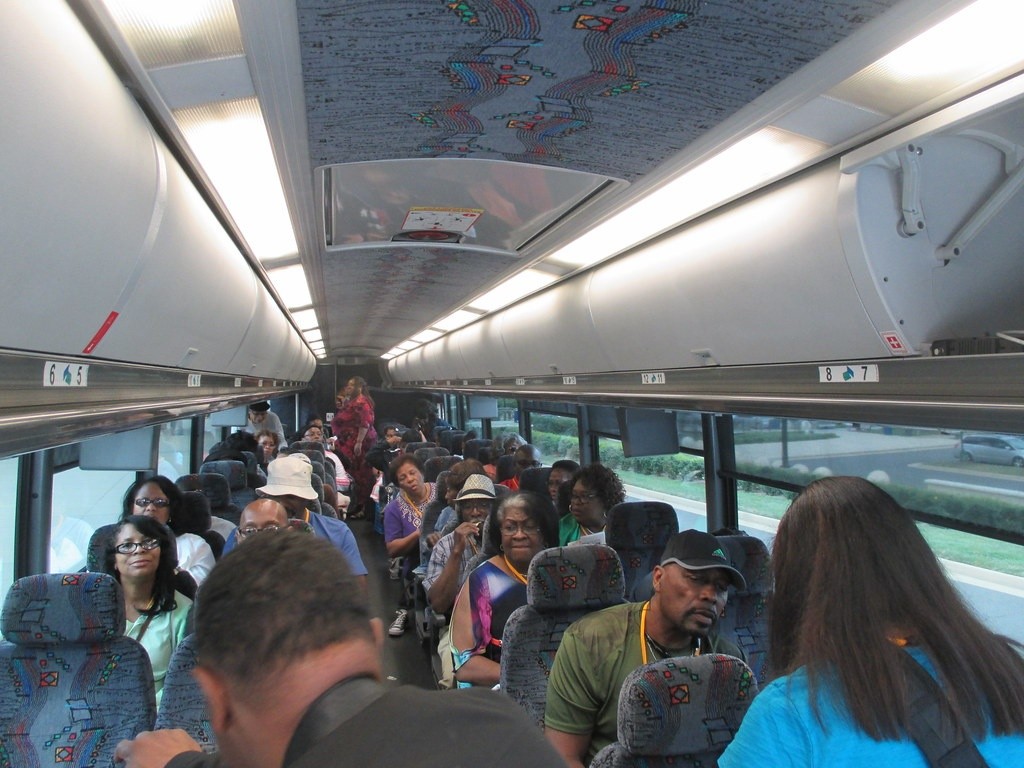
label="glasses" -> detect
[498,518,543,537]
[509,445,528,454]
[303,430,325,439]
[458,501,494,516]
[237,525,281,537]
[515,459,542,470]
[251,412,268,417]
[567,489,599,505]
[111,538,159,555]
[134,496,170,508]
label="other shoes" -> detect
[349,503,365,519]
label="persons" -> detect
[231,401,288,452]
[235,498,294,546]
[559,463,625,548]
[174,474,238,562]
[122,475,216,587]
[301,424,350,492]
[640,601,701,665]
[255,429,279,464]
[544,530,747,768]
[330,375,378,520]
[113,528,572,768]
[306,416,347,468]
[448,491,560,692]
[102,515,193,714]
[223,457,368,595]
[717,476,1024,768]
[366,426,579,636]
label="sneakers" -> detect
[387,610,410,636]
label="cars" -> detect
[951,434,1024,467]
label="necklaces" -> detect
[146,597,154,608]
[305,508,309,522]
[504,554,527,584]
[513,479,518,489]
[580,524,606,536]
[469,538,478,554]
[405,484,430,517]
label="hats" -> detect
[250,401,272,412]
[295,423,320,441]
[660,530,747,592]
[455,474,499,502]
[255,456,319,501]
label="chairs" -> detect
[0,406,772,768]
